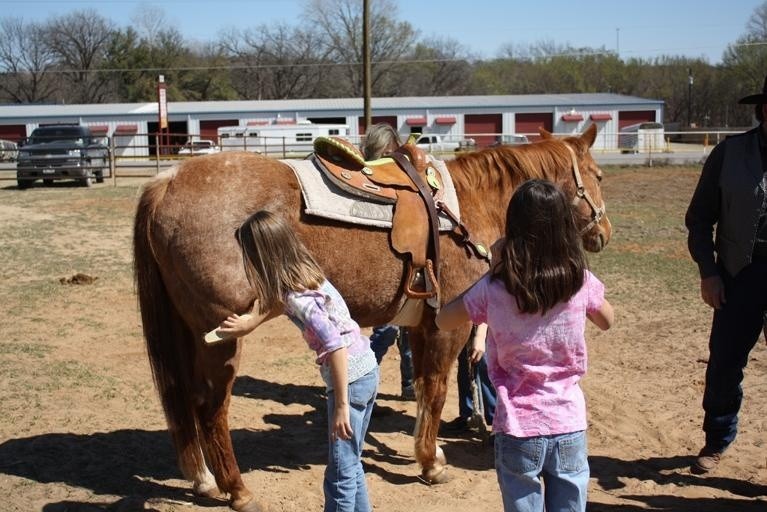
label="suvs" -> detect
[15,125,106,188]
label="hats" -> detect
[738,75,766,105]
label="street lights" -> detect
[686,66,694,127]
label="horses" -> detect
[133,121,613,512]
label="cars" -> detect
[485,135,530,149]
[179,140,220,156]
[0,140,17,162]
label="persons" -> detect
[215,210,381,512]
[359,124,416,399]
[685,72,767,472]
[441,314,500,444]
[434,178,615,512]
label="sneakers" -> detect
[446,415,467,428]
[694,444,723,472]
[401,384,419,400]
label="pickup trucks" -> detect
[403,133,475,152]
